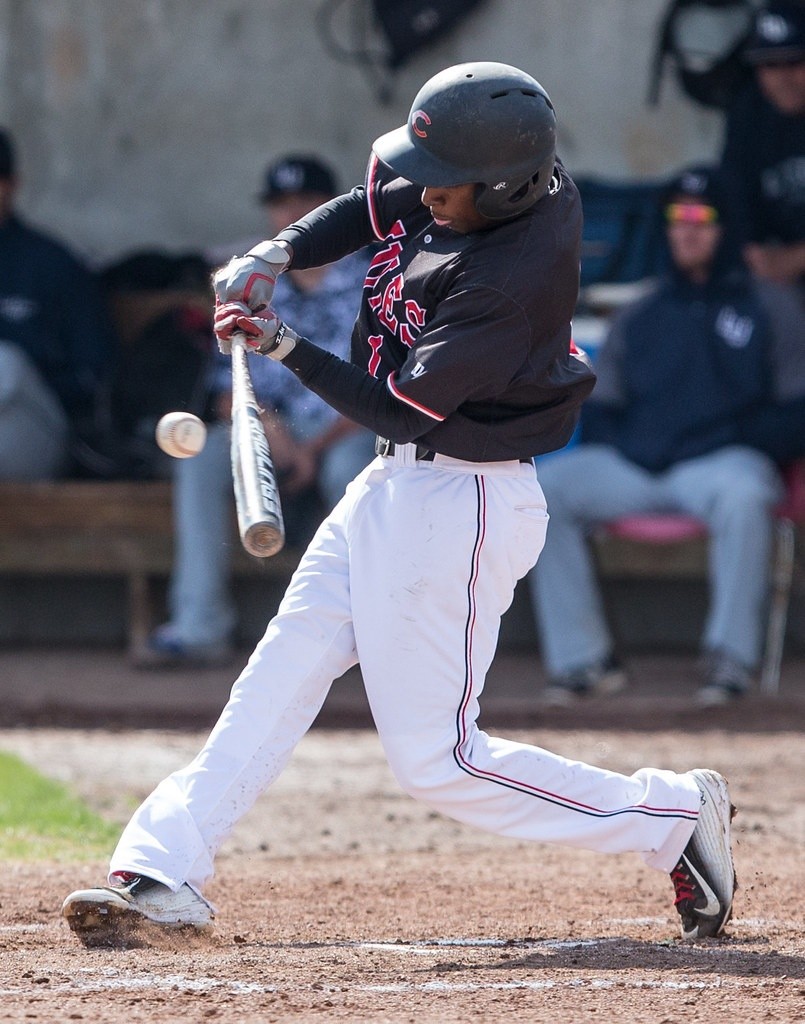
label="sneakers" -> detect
[669,769,737,942]
[61,870,216,947]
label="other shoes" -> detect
[134,619,227,668]
[689,659,754,711]
[542,666,634,710]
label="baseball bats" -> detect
[226,328,288,559]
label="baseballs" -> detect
[155,411,208,460]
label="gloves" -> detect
[211,240,290,314]
[213,291,300,363]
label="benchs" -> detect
[0,481,805,673]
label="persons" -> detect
[723,14,805,403]
[527,166,804,705]
[0,126,90,483]
[63,62,740,941]
[156,154,375,662]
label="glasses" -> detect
[657,201,720,226]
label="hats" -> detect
[256,154,334,203]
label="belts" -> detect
[374,440,533,465]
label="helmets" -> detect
[650,165,752,249]
[739,8,805,70]
[371,62,557,220]
